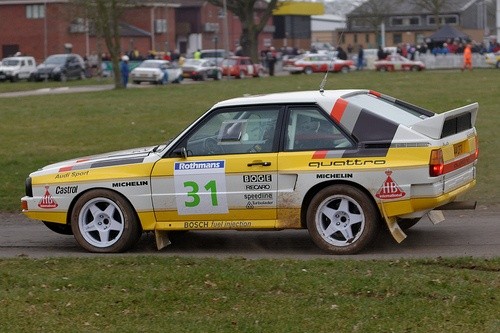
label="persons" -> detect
[81,33,500,87]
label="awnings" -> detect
[424,24,461,39]
[90,22,150,36]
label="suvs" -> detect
[193,49,234,65]
[0,56,36,83]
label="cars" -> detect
[317,50,338,57]
[221,56,267,79]
[20,30,479,255]
[312,42,336,52]
[131,59,184,85]
[384,47,401,56]
[484,50,500,69]
[374,54,425,71]
[281,51,357,75]
[352,48,379,69]
[29,54,88,82]
[179,59,223,82]
